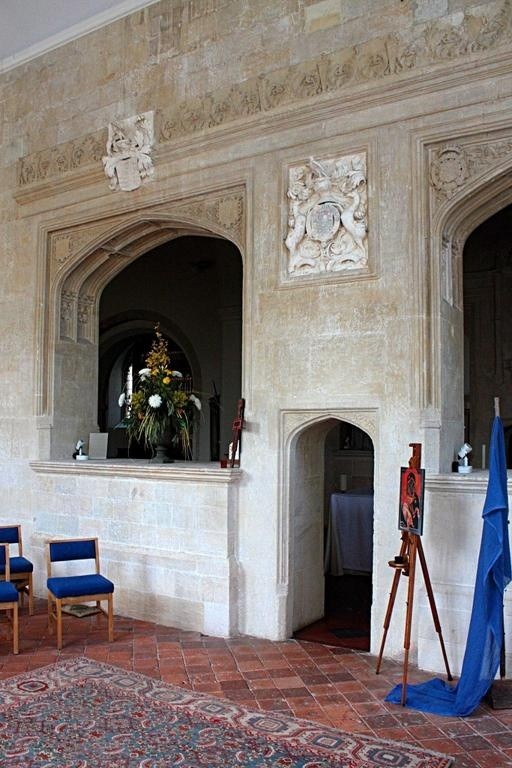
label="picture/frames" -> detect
[398,466,426,536]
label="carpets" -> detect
[0,655,457,768]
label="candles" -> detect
[341,473,347,491]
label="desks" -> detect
[323,486,374,596]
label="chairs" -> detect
[0,524,115,656]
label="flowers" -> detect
[117,321,202,463]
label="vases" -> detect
[149,429,175,464]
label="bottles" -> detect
[334,471,353,494]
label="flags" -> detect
[386,409,511,718]
